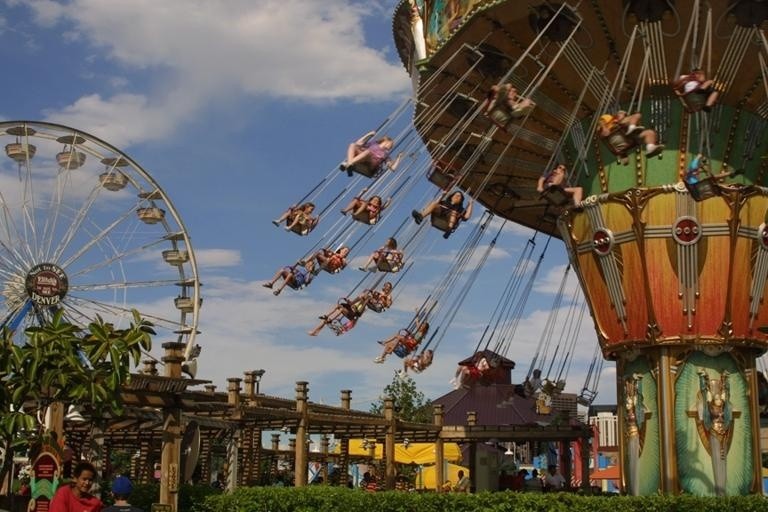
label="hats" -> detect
[111,477,132,495]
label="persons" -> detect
[340,188,391,217]
[529,468,544,490]
[511,369,541,398]
[263,246,350,296]
[512,468,529,491]
[544,464,567,490]
[366,475,376,490]
[456,469,471,493]
[597,110,664,158]
[687,153,756,193]
[273,202,319,230]
[339,131,404,177]
[101,476,144,511]
[411,190,473,239]
[679,69,720,112]
[404,350,432,372]
[360,471,375,493]
[358,237,403,274]
[450,352,489,390]
[537,164,585,212]
[47,460,104,512]
[376,322,429,365]
[308,282,393,338]
[487,82,532,119]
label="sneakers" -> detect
[319,315,332,323]
[341,209,356,217]
[375,359,383,364]
[511,105,530,117]
[548,184,558,193]
[495,85,506,102]
[297,260,305,266]
[263,282,281,296]
[341,162,354,177]
[695,88,705,95]
[701,105,712,113]
[312,271,319,276]
[626,127,645,139]
[645,146,664,159]
[378,340,393,355]
[358,266,377,273]
[412,210,423,223]
[571,207,584,214]
[443,227,451,239]
[450,376,461,389]
[272,218,290,232]
